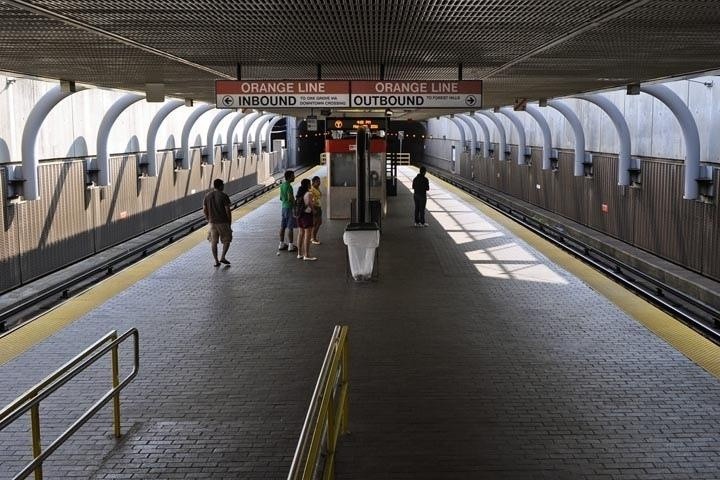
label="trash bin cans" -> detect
[343,222,383,285]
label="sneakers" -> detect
[414,222,430,228]
[297,255,319,261]
[310,238,321,245]
[278,242,297,252]
[214,258,231,268]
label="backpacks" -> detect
[292,195,306,219]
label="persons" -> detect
[294,179,318,261]
[411,166,430,228]
[277,170,297,252]
[202,178,233,267]
[309,176,323,245]
